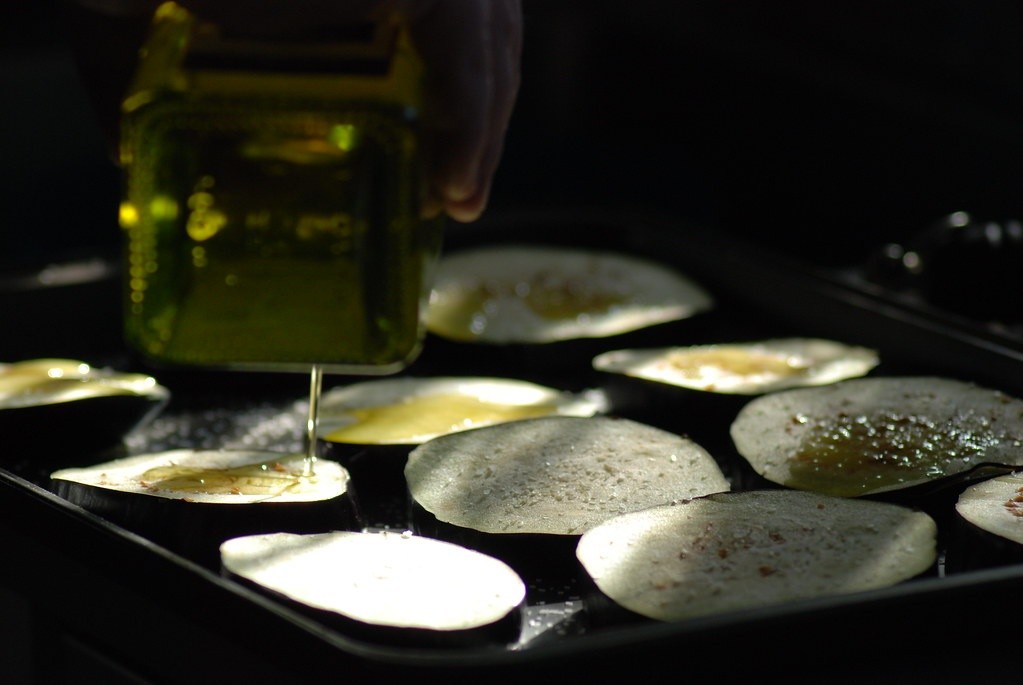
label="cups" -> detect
[110,2,447,373]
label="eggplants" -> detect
[1,247,1023,652]
